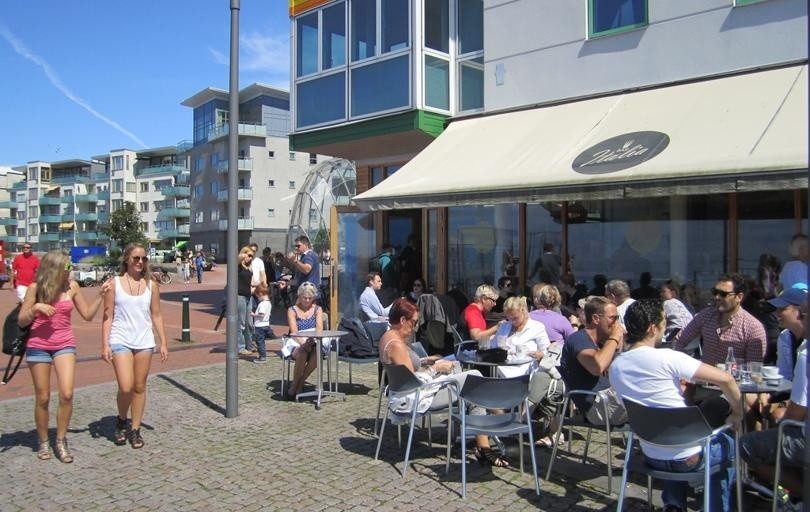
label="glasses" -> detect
[486,297,496,302]
[413,284,422,286]
[599,314,620,321]
[710,287,740,298]
[411,318,419,327]
[23,247,30,250]
[505,284,513,288]
[130,255,148,264]
[63,265,72,271]
[295,244,303,248]
[246,252,254,259]
[303,282,314,287]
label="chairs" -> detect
[542,343,614,497]
[445,371,542,500]
[741,418,810,511]
[360,303,416,345]
[616,393,745,512]
[448,323,482,356]
[373,363,460,481]
[279,312,332,399]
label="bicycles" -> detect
[150,265,173,284]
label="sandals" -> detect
[54,438,73,463]
[535,432,565,448]
[128,424,145,449]
[113,415,128,445]
[38,440,53,462]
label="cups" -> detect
[736,358,745,382]
[764,366,779,377]
[740,364,751,383]
[715,363,725,370]
[750,362,762,382]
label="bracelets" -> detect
[606,338,623,344]
[24,308,35,319]
[778,400,786,407]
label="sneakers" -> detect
[247,343,257,352]
[777,484,790,504]
[253,358,266,364]
[239,349,252,355]
[776,500,804,512]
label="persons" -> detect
[407,279,425,300]
[285,282,323,398]
[279,235,320,305]
[779,235,808,286]
[746,289,801,433]
[491,277,516,319]
[532,282,547,308]
[375,297,511,467]
[400,234,418,288]
[102,242,169,448]
[747,287,780,359]
[671,274,766,409]
[604,279,636,351]
[173,248,206,284]
[16,251,112,464]
[529,242,562,283]
[11,243,39,302]
[740,302,810,498]
[527,284,573,359]
[569,299,589,328]
[658,283,701,353]
[452,284,506,356]
[224,246,258,361]
[250,245,276,341]
[262,246,275,283]
[377,244,396,303]
[485,297,550,414]
[557,271,586,313]
[358,271,401,324]
[559,297,630,425]
[609,298,736,511]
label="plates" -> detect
[763,375,785,381]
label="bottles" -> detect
[725,347,735,377]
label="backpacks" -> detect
[370,258,381,274]
[383,256,401,288]
[1,300,31,356]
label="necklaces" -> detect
[125,275,141,295]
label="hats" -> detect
[766,287,810,308]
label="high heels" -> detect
[295,391,304,402]
[285,388,294,401]
[475,447,511,468]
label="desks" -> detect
[700,363,793,394]
[456,347,535,380]
[289,329,349,411]
